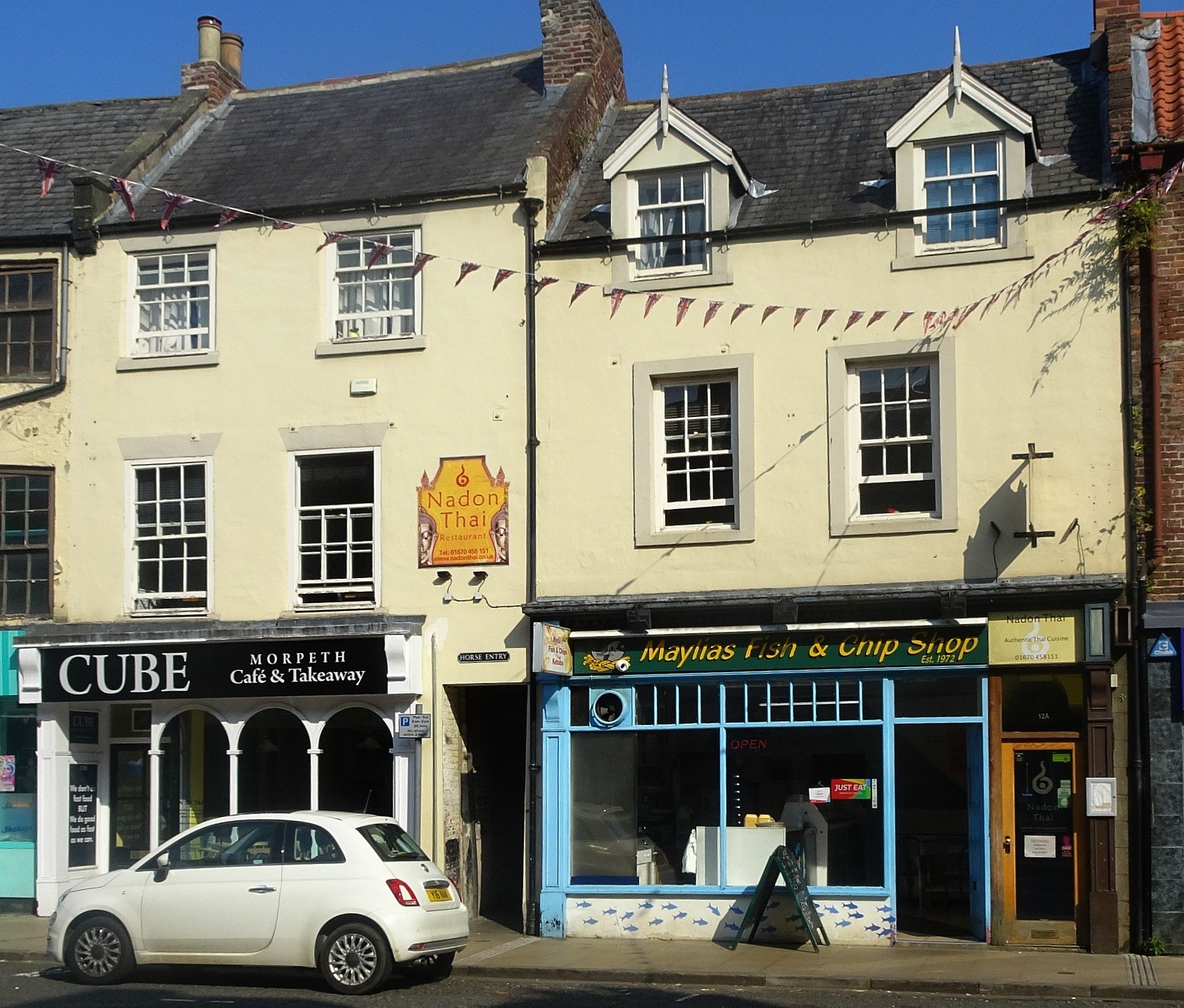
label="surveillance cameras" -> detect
[616,660,629,671]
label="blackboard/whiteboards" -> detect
[729,844,831,954]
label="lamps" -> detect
[772,597,799,623]
[474,571,488,578]
[626,604,651,629]
[437,571,451,578]
[941,589,966,618]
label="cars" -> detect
[46,808,470,996]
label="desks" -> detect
[695,826,802,886]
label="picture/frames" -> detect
[1086,777,1117,816]
